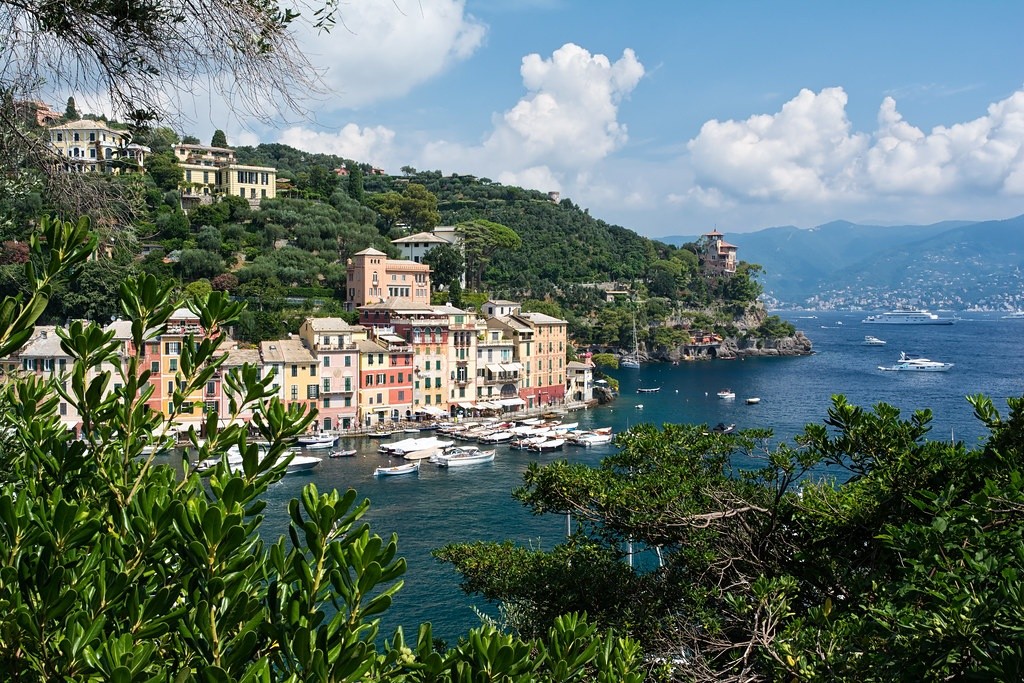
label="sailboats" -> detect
[620,313,641,369]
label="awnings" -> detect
[416,398,526,417]
[172,419,248,432]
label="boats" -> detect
[636,387,661,393]
[307,439,334,450]
[715,388,736,399]
[877,351,955,372]
[376,459,422,475]
[861,334,888,345]
[861,305,961,324]
[191,442,324,479]
[328,450,357,458]
[297,429,341,445]
[378,414,616,468]
[141,445,174,455]
[745,397,761,405]
